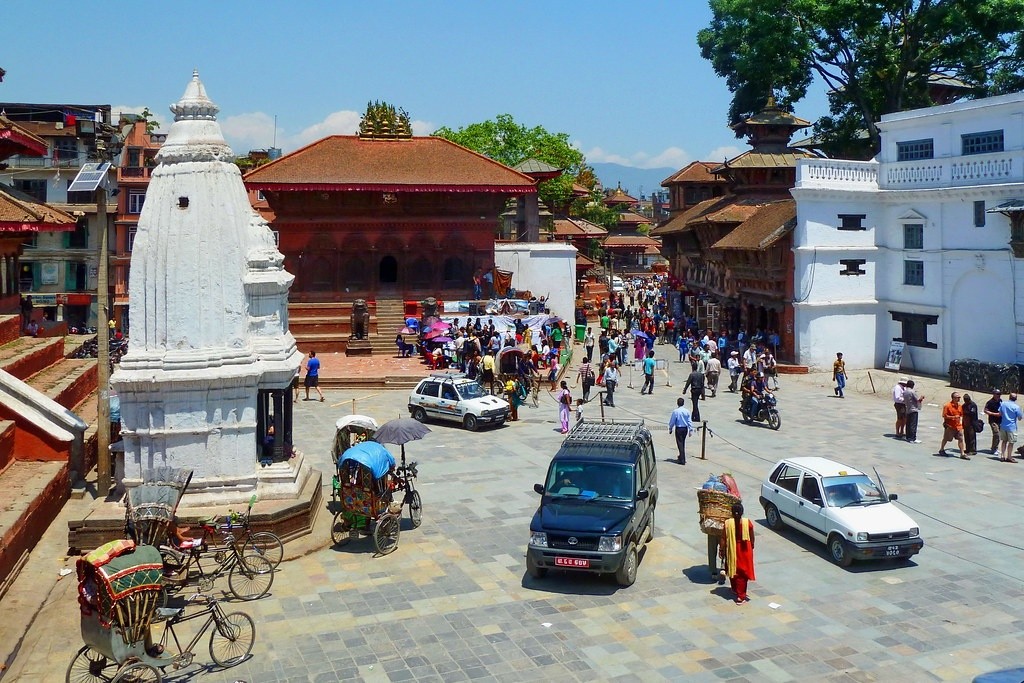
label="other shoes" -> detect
[964,450,976,455]
[1000,456,1019,463]
[834,387,845,399]
[752,413,758,420]
[302,397,310,401]
[720,571,726,584]
[907,438,921,443]
[895,434,906,439]
[712,574,717,582]
[960,454,970,460]
[319,397,325,402]
[992,449,1001,455]
[939,450,949,457]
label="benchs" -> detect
[502,365,517,376]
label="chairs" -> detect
[415,343,444,370]
[396,342,408,357]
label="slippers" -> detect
[737,598,746,605]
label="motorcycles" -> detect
[71,335,129,374]
[738,389,781,430]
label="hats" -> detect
[898,377,908,384]
[730,351,738,355]
[750,344,757,349]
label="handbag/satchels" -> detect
[764,362,778,375]
[974,419,985,432]
[586,377,595,385]
[561,390,572,404]
[463,337,478,355]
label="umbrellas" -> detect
[430,323,451,328]
[632,331,648,338]
[423,330,443,339]
[373,414,431,467]
[434,337,452,341]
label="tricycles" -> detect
[331,441,422,554]
[330,415,380,515]
[492,347,539,408]
[142,466,284,574]
[65,539,255,683]
[124,486,275,601]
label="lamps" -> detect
[111,189,121,196]
[75,119,96,138]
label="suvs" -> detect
[408,373,511,431]
[526,417,659,586]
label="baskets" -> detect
[698,489,742,536]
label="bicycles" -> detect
[485,296,518,315]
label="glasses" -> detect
[994,393,1002,395]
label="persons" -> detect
[962,393,978,455]
[291,364,301,402]
[550,472,582,493]
[109,317,116,337]
[893,377,907,437]
[845,484,880,502]
[114,328,122,339]
[832,352,848,398]
[22,295,33,331]
[394,268,780,463]
[719,502,755,604]
[43,313,48,322]
[939,392,970,460]
[998,393,1022,463]
[983,389,1003,455]
[27,319,38,333]
[903,381,924,442]
[53,146,59,168]
[304,351,325,402]
[707,472,741,580]
[606,471,627,498]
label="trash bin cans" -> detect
[575,325,585,342]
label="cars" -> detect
[613,282,625,292]
[759,457,923,566]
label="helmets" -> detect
[756,372,764,377]
